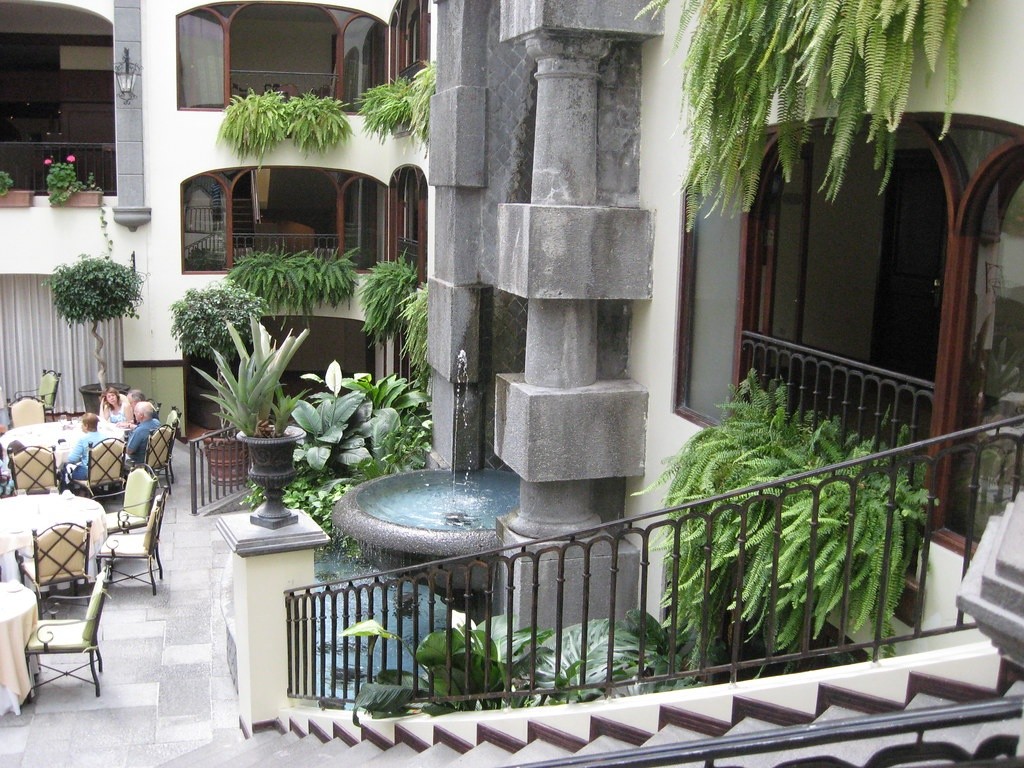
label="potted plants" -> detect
[168,279,271,487]
[189,314,315,531]
[215,61,436,173]
[41,252,146,416]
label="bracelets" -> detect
[128,423,131,428]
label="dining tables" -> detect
[0,582,39,717]
[0,419,132,475]
[0,493,108,593]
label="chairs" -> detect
[15,519,93,620]
[123,424,175,496]
[89,460,161,561]
[67,436,128,505]
[14,369,61,422]
[95,485,170,597]
[24,556,114,704]
[165,406,182,485]
[7,396,47,428]
[6,445,63,495]
[144,399,162,418]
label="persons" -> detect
[100,388,131,422]
[115,390,160,429]
[0,444,15,497]
[121,401,162,475]
[61,411,111,496]
[8,439,61,486]
